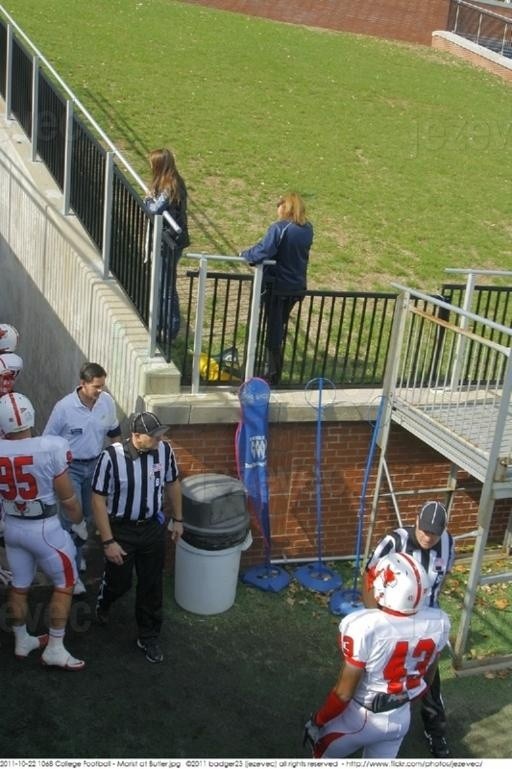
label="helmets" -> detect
[367,549,433,617]
[1,392,37,436]
[1,351,24,377]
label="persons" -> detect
[300,551,452,761]
[41,361,123,595]
[363,500,457,758]
[239,192,314,392]
[0,393,89,669]
[0,353,24,397]
[142,148,191,342]
[91,411,185,664]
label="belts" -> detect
[370,688,410,715]
[120,519,153,527]
[71,456,101,464]
[1,500,59,520]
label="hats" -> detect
[417,499,449,537]
[130,411,171,438]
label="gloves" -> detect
[302,711,324,754]
[67,520,89,549]
[0,566,14,587]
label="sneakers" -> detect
[40,652,86,669]
[93,585,112,626]
[13,633,49,659]
[137,639,163,667]
[424,729,450,757]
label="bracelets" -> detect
[172,518,184,522]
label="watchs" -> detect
[99,538,117,546]
